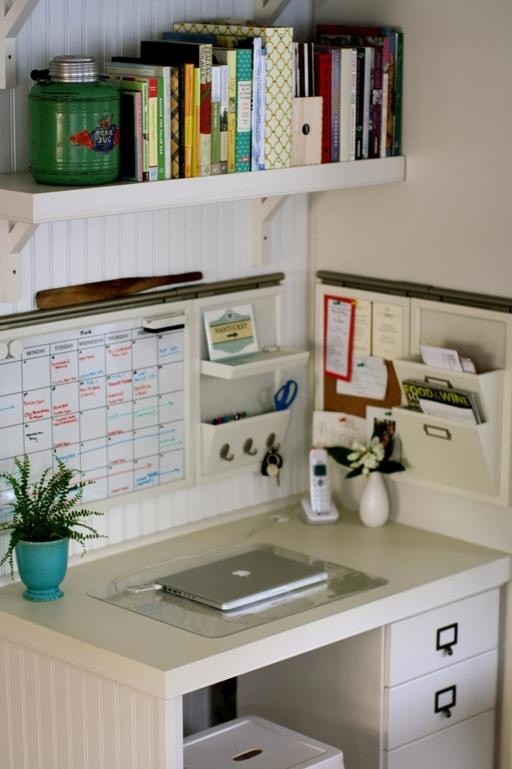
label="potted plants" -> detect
[0,453,111,605]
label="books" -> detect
[95,17,401,180]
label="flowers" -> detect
[325,418,402,477]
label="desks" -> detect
[2,500,512,768]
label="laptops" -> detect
[157,550,327,615]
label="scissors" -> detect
[274,380,298,411]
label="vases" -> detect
[356,472,392,529]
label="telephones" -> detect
[298,448,340,526]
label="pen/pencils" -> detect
[207,412,247,425]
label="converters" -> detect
[272,511,292,527]
